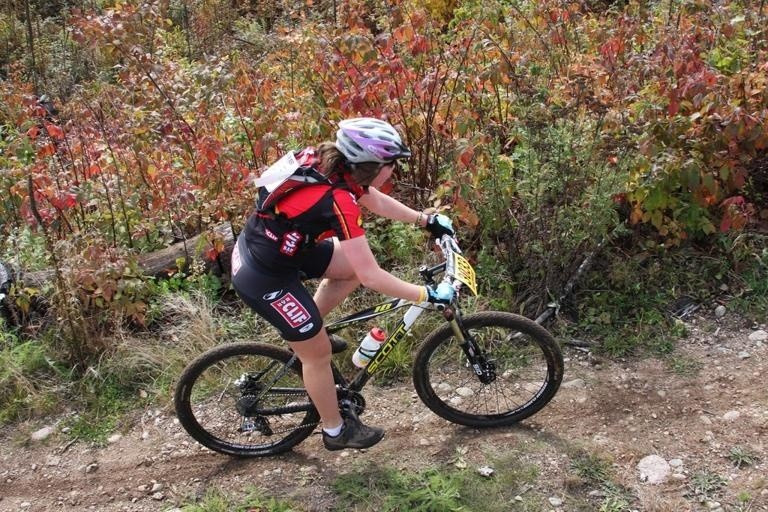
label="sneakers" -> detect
[319,415,386,452]
[286,330,348,355]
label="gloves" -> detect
[419,283,457,308]
[425,212,456,241]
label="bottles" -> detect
[351,327,386,369]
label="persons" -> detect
[227,117,457,451]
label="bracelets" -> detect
[415,212,423,226]
[415,285,425,305]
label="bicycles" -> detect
[174,234,564,458]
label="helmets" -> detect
[332,115,413,165]
